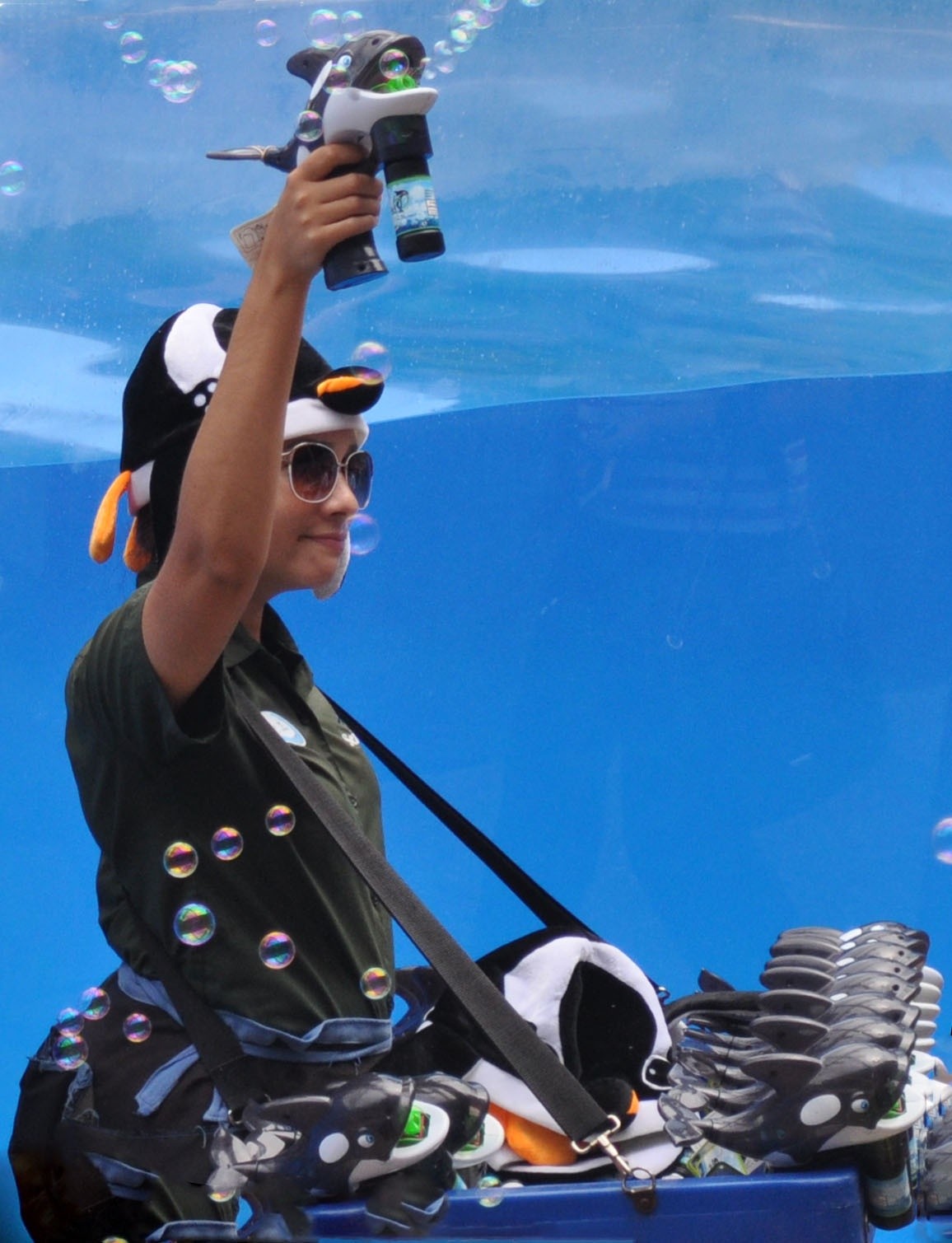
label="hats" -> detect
[89,303,386,573]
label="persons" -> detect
[61,140,395,1132]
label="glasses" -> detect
[273,442,376,509]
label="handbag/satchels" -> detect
[426,928,679,1172]
